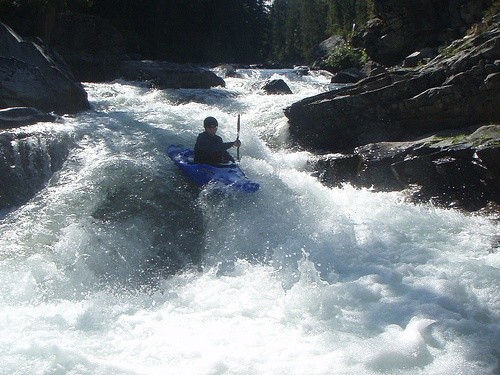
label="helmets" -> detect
[203,117,220,128]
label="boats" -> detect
[167,143,260,193]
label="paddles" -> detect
[236,114,241,162]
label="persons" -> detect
[194,117,241,163]
